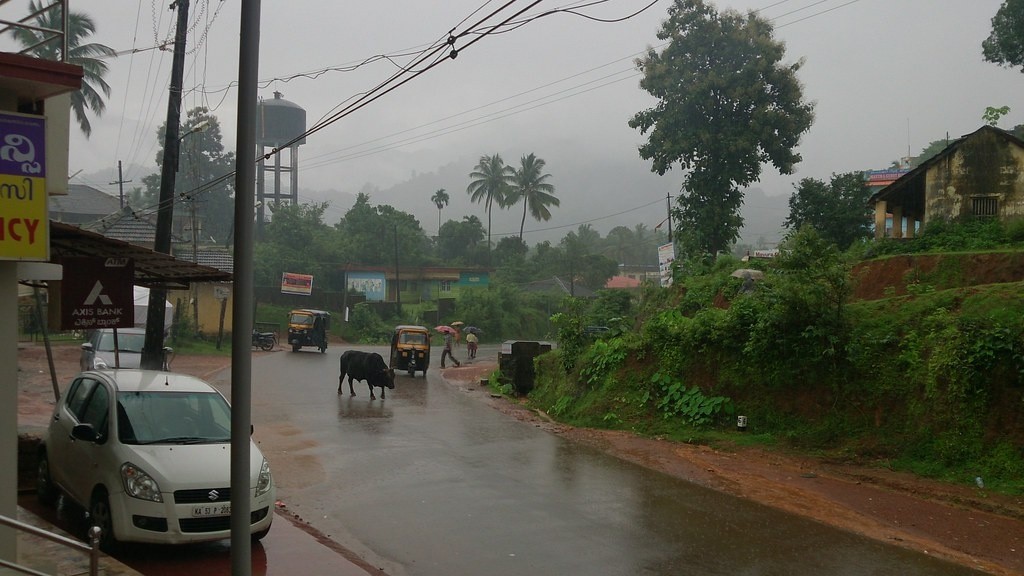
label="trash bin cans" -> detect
[502,340,540,391]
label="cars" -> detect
[78,324,170,372]
[44,367,273,551]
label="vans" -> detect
[587,326,608,333]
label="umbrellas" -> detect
[462,327,476,330]
[451,322,465,325]
[433,326,456,334]
[729,269,762,279]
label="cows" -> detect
[337,350,396,400]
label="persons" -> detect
[454,325,460,347]
[742,272,753,292]
[439,331,460,367]
[466,331,478,358]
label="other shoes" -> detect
[473,356,476,357]
[457,362,460,367]
[440,366,445,369]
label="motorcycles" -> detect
[286,309,330,353]
[389,326,432,377]
[252,328,278,351]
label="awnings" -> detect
[18,218,233,290]
